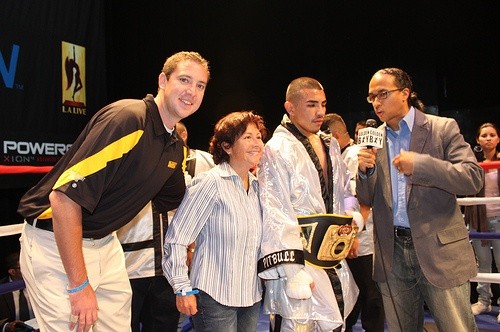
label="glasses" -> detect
[366,88,403,103]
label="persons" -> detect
[17,51,212,332]
[174,122,217,184]
[160,112,274,332]
[115,142,195,332]
[256,77,362,332]
[354,68,487,331]
[0,252,37,332]
[456,122,500,324]
[319,113,388,331]
[353,121,367,141]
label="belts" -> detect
[393,226,411,238]
[26,215,111,240]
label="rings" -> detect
[69,314,80,326]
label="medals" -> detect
[295,213,358,269]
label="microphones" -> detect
[357,119,383,174]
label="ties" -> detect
[19,289,30,321]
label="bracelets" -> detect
[175,288,199,298]
[65,279,88,293]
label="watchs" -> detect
[189,247,194,253]
